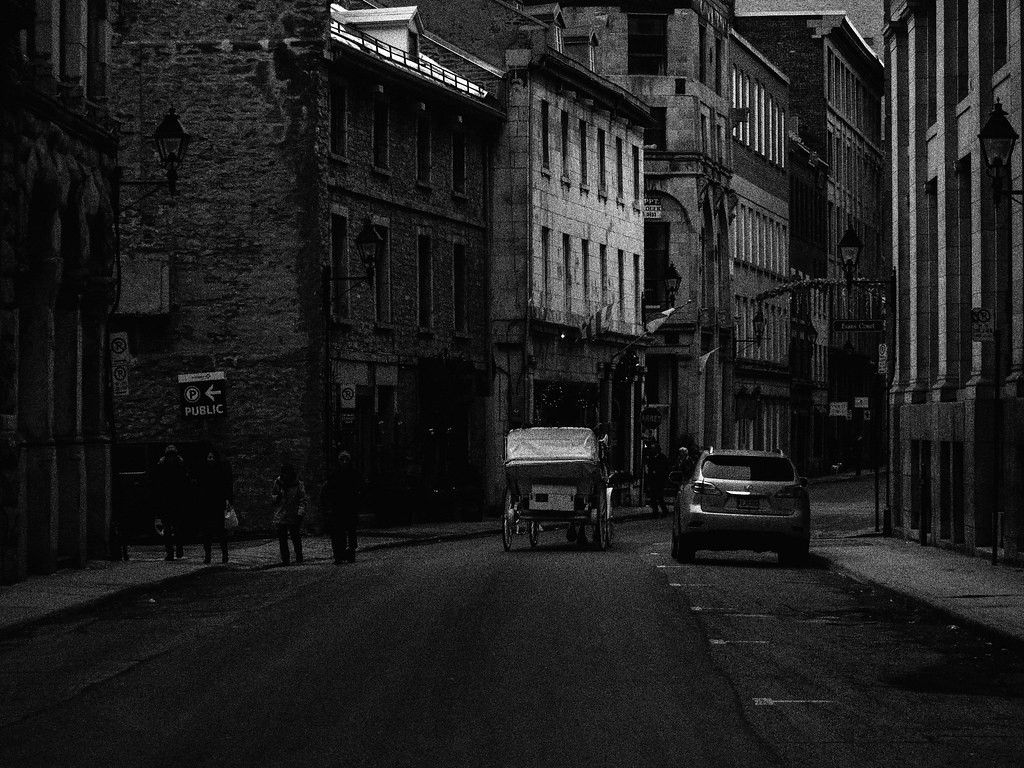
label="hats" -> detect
[338,450,352,462]
[164,445,179,455]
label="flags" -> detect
[581,301,614,341]
[644,307,675,333]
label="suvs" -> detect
[667,446,813,567]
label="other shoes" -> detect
[332,557,344,565]
[203,558,211,564]
[176,554,184,558]
[282,560,291,566]
[165,556,174,561]
[296,559,304,566]
[346,551,357,563]
[222,559,228,564]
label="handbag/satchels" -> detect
[223,499,239,530]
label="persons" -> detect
[154,446,195,560]
[274,461,309,567]
[320,449,367,565]
[196,448,234,565]
[647,443,669,518]
[673,447,697,482]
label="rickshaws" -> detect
[500,426,618,551]
